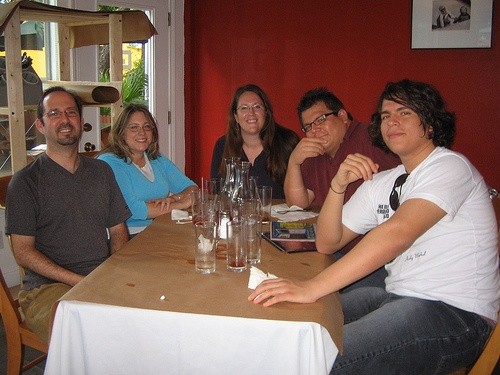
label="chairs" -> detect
[0,175,50,375]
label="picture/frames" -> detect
[410,0,494,50]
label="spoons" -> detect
[278,208,311,214]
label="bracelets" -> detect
[170,193,182,202]
[330,183,347,194]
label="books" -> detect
[257,222,317,254]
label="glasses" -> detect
[235,103,266,113]
[125,124,153,132]
[389,173,410,211]
[42,108,81,119]
[302,112,338,134]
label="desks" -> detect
[44,195,343,375]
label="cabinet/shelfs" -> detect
[0,5,124,175]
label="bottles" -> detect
[217,157,240,217]
[230,162,254,228]
[218,211,231,238]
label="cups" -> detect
[243,215,261,264]
[256,186,272,224]
[206,178,224,201]
[249,176,258,192]
[195,221,217,275]
[226,220,248,272]
[241,199,261,216]
[202,201,221,225]
[191,189,208,224]
[215,239,235,259]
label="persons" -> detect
[283,87,403,294]
[210,84,302,199]
[248,79,500,375]
[6,87,133,347]
[95,104,199,241]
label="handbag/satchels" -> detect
[0,52,43,106]
